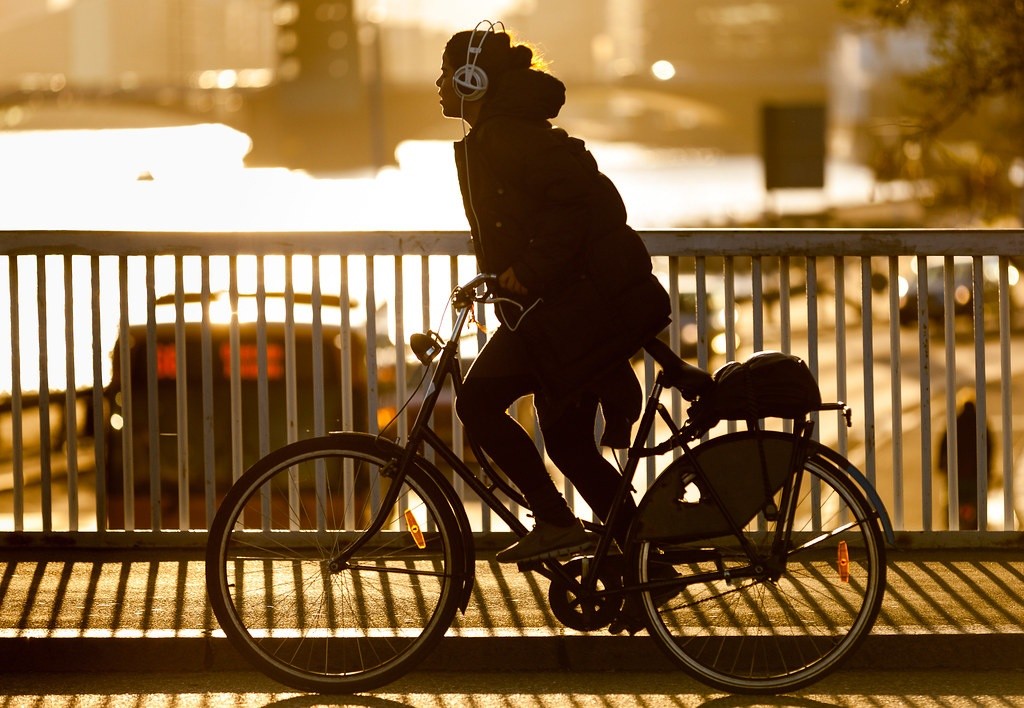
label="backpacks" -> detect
[627,351,822,459]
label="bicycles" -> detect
[205,271,896,696]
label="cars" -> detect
[92,283,387,541]
[899,253,1024,330]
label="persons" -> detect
[436,31,688,635]
[938,400,996,530]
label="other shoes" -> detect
[495,520,595,565]
[608,569,689,637]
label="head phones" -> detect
[452,19,506,101]
